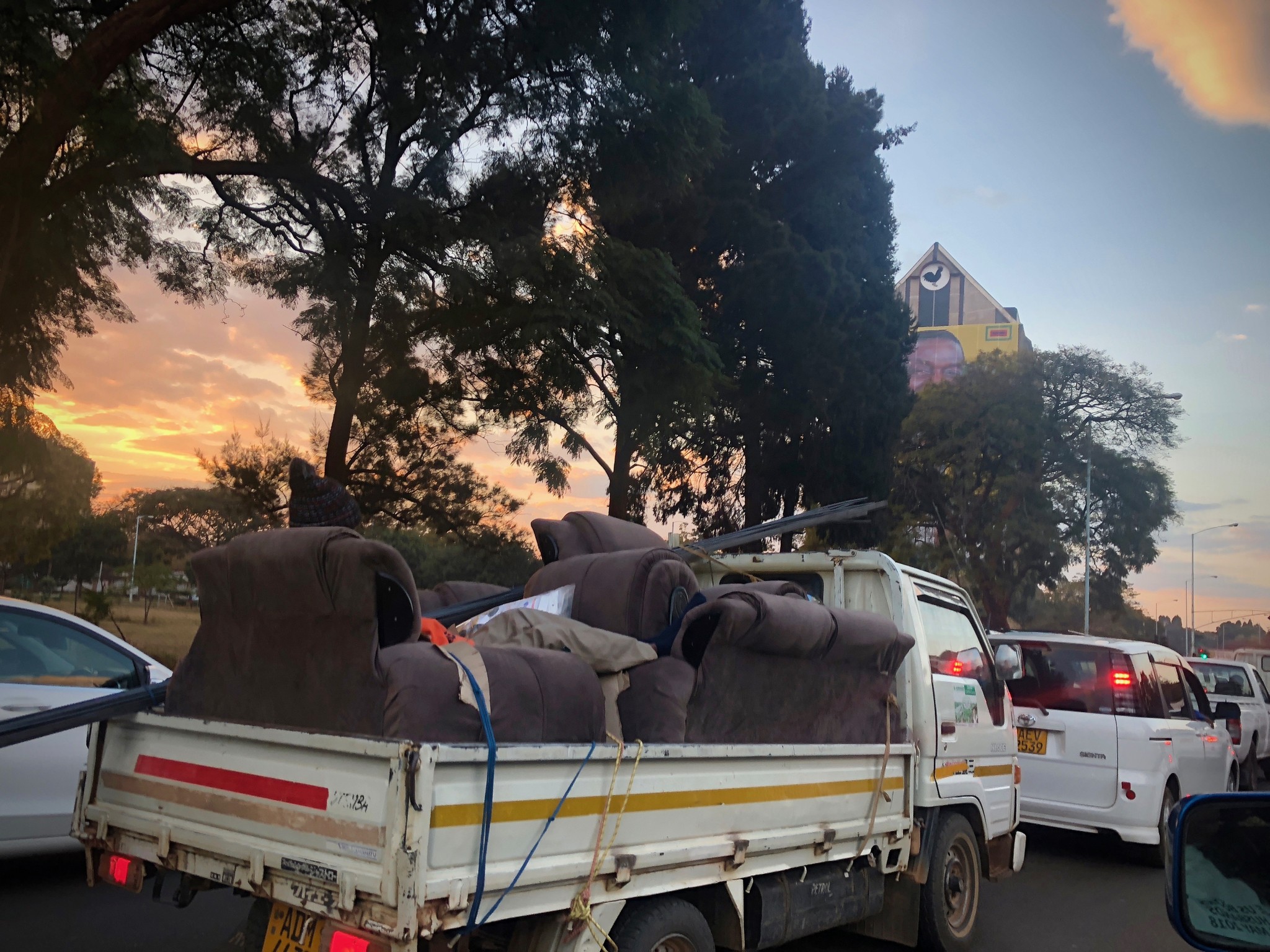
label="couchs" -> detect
[161,509,919,745]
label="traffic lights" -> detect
[1198,648,1210,659]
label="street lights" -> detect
[1156,599,1178,635]
[1185,575,1218,656]
[1192,523,1238,655]
[129,515,154,604]
[1084,392,1182,634]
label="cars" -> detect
[0,595,175,859]
[1233,647,1270,699]
[1181,656,1270,782]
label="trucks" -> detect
[67,548,1027,951]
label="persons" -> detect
[907,328,965,396]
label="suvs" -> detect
[986,631,1241,869]
